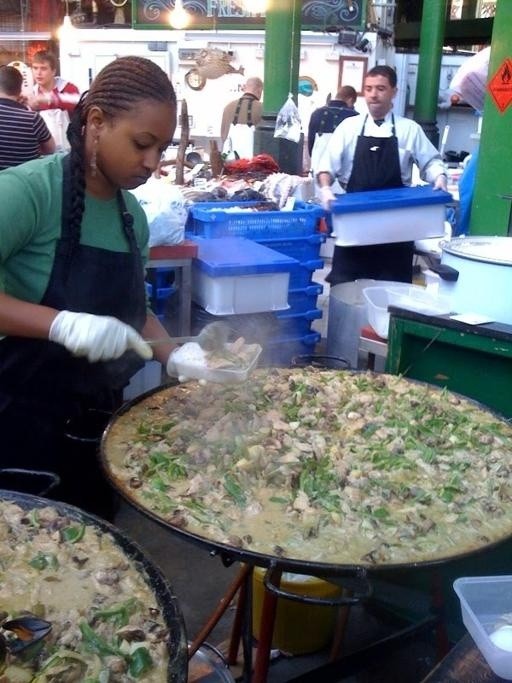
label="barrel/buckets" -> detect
[246,567,352,660]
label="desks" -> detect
[143,239,195,339]
[386,302,511,426]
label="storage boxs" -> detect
[329,185,453,248]
[361,283,426,340]
[191,237,300,316]
[452,574,512,680]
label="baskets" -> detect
[184,198,327,363]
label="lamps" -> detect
[56,0,79,45]
[168,0,193,28]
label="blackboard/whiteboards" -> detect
[131,0,367,32]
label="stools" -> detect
[358,324,389,375]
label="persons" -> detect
[313,65,449,283]
[27,51,79,149]
[307,85,360,197]
[220,78,263,161]
[0,56,207,523]
[0,66,55,171]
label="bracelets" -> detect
[47,94,51,108]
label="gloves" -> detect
[319,185,338,211]
[164,347,190,382]
[47,309,154,362]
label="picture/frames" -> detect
[337,55,368,96]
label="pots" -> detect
[429,235,512,324]
